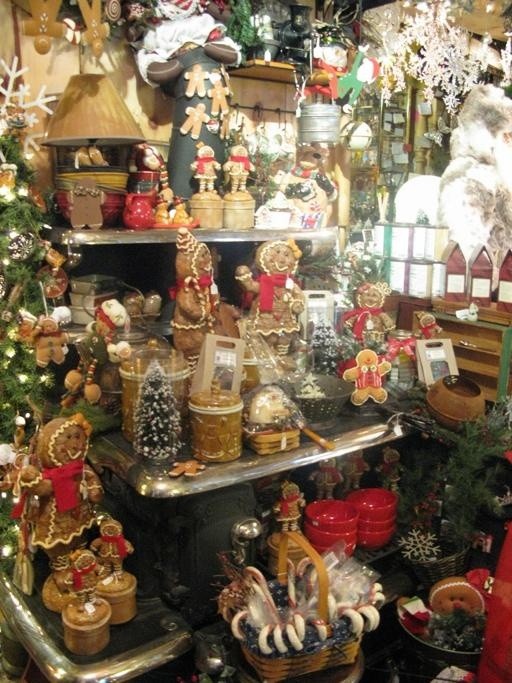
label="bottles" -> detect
[188,192,257,234]
[186,379,244,462]
[280,4,314,61]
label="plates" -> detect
[67,271,120,327]
[397,589,483,657]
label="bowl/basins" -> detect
[55,164,127,228]
[302,488,398,558]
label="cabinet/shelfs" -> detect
[0,59,415,683]
[391,295,512,402]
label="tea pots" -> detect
[123,190,157,229]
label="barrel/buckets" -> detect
[298,94,342,144]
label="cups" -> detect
[117,346,190,465]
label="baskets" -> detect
[243,429,301,455]
[406,536,472,589]
[231,532,365,683]
